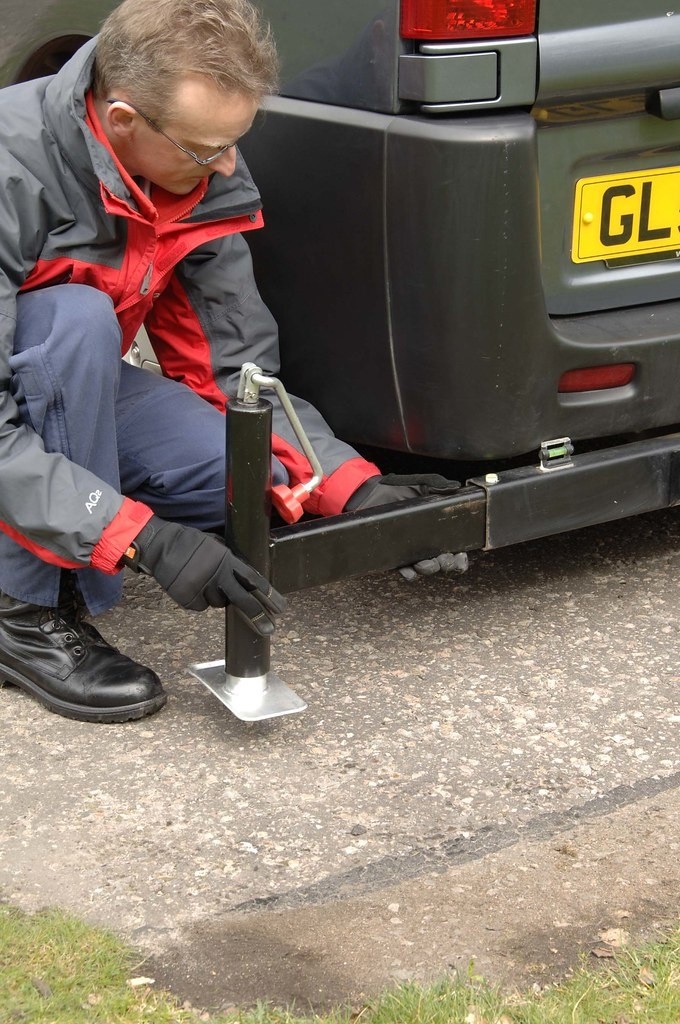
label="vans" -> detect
[0,0,680,723]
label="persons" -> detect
[0,0,468,724]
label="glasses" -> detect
[107,97,235,167]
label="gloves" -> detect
[123,513,287,635]
[345,474,470,580]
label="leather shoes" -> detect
[0,588,168,723]
